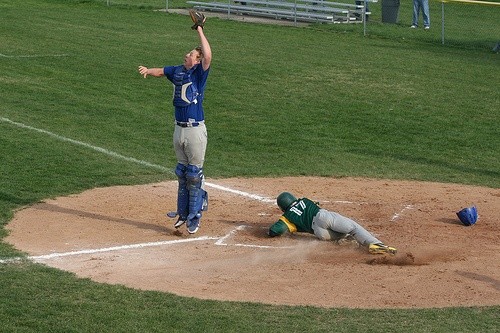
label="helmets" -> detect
[456,207,477,225]
[277,191,297,212]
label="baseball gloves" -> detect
[189,9,207,29]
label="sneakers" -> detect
[337,238,359,248]
[188,216,200,234]
[369,244,397,255]
[175,216,186,228]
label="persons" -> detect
[139,10,212,234]
[269,192,397,254]
[355,0,368,22]
[410,0,430,29]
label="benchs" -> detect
[186,0,378,24]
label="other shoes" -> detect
[409,25,416,28]
[424,26,429,29]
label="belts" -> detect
[313,210,319,224]
[177,122,199,128]
